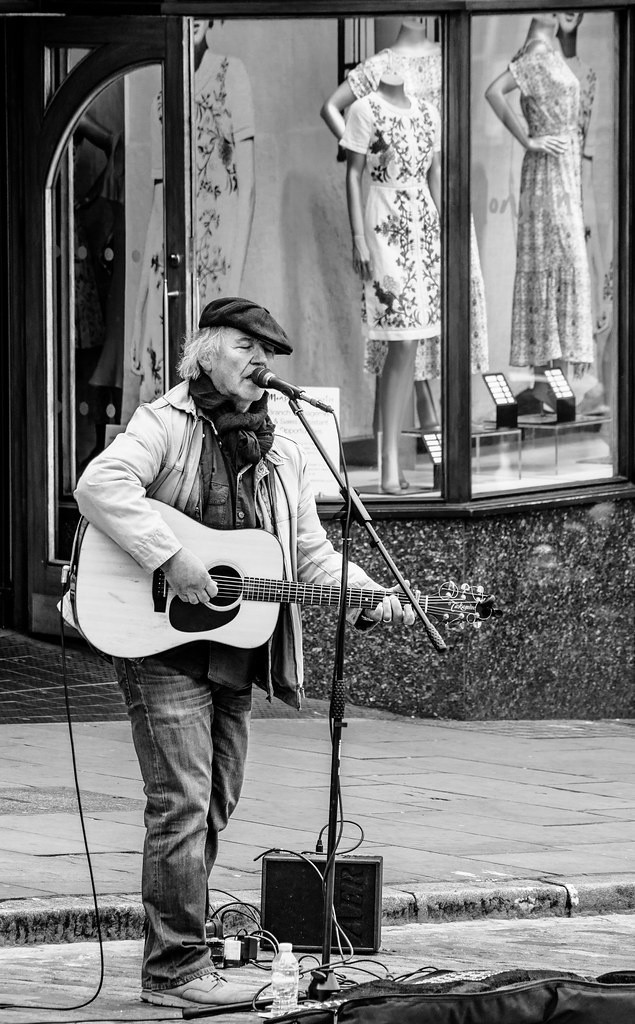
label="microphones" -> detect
[251,366,335,413]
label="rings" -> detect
[383,619,391,623]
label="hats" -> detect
[199,297,293,355]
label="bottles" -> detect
[270,942,299,1019]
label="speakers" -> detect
[260,853,383,953]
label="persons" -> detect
[484,17,595,412]
[339,73,441,496]
[510,10,610,335]
[320,22,489,436]
[74,297,414,1012]
[128,19,256,400]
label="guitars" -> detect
[70,495,497,660]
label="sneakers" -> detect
[141,973,265,1010]
